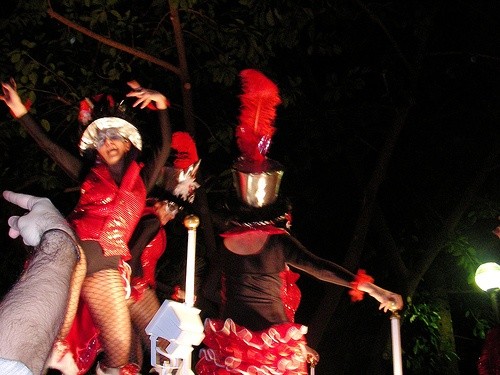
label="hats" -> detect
[220,70,285,227]
[77,87,143,157]
[156,131,203,212]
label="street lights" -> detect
[476,262,500,345]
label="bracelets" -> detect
[41,228,81,262]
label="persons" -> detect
[0,83,172,375]
[0,70,403,375]
